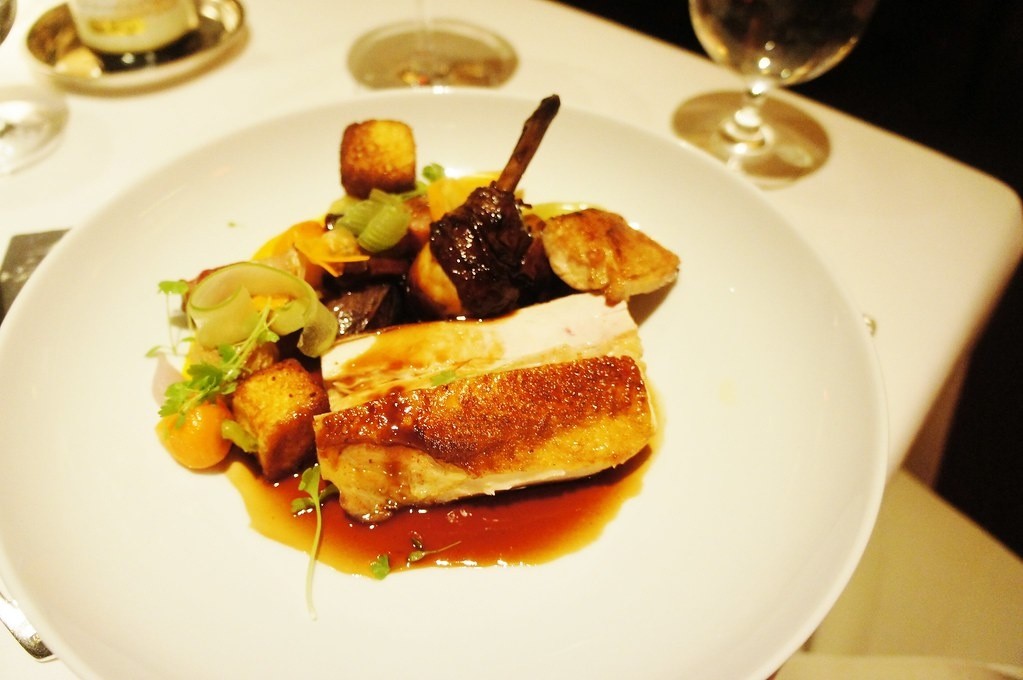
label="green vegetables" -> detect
[148,282,462,617]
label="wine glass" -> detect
[0,0,68,174]
[673,0,876,182]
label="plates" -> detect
[1,87,888,680]
[349,19,516,92]
[19,1,244,99]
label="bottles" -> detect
[61,0,199,74]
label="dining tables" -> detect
[0,0,1023,476]
